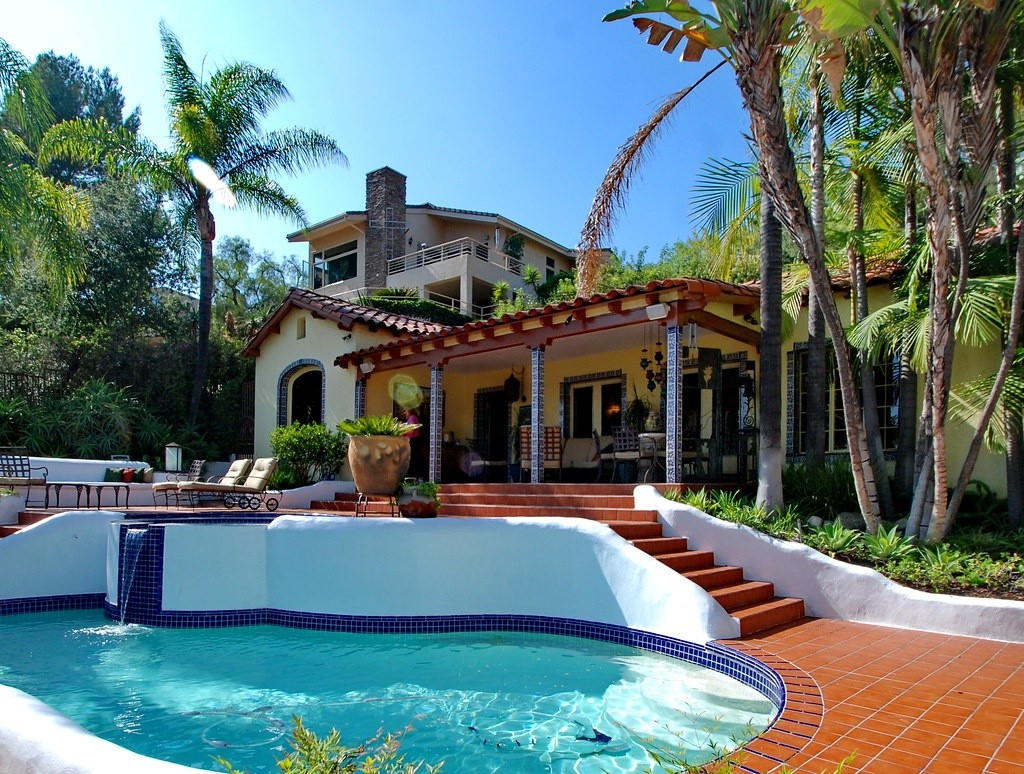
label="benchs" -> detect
[562,436,614,482]
[637,433,712,483]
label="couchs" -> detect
[0,455,172,507]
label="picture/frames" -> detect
[392,382,445,429]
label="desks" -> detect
[440,443,468,483]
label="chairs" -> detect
[0,446,48,510]
[152,458,283,511]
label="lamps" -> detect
[744,313,759,327]
[646,303,671,321]
[504,364,526,403]
[565,314,573,326]
[409,236,413,245]
[483,235,489,241]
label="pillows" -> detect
[133,468,144,482]
[104,467,125,482]
[144,468,155,482]
[122,468,136,482]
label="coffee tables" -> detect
[45,481,130,510]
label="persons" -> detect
[398,403,425,477]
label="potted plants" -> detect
[622,378,653,431]
[335,414,423,494]
[0,487,22,526]
[388,479,442,519]
